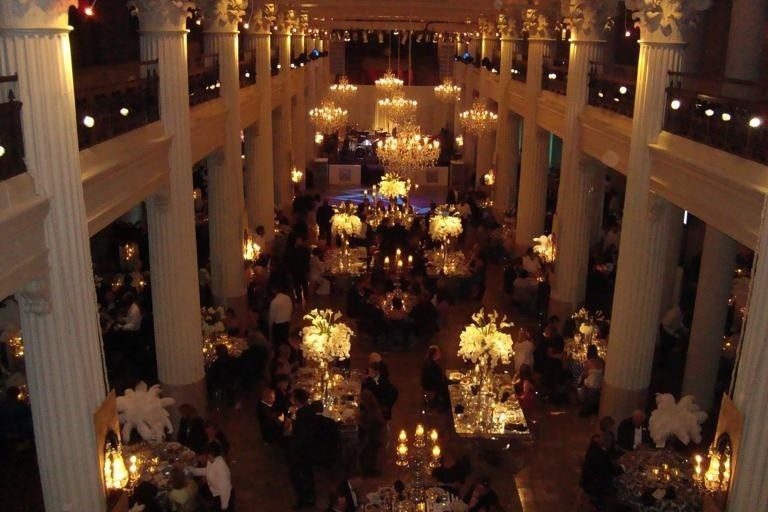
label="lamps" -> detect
[309,0,500,177]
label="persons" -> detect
[1,47,734,512]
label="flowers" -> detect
[427,205,465,243]
[378,174,411,198]
[299,307,354,370]
[457,308,515,368]
[328,200,362,238]
